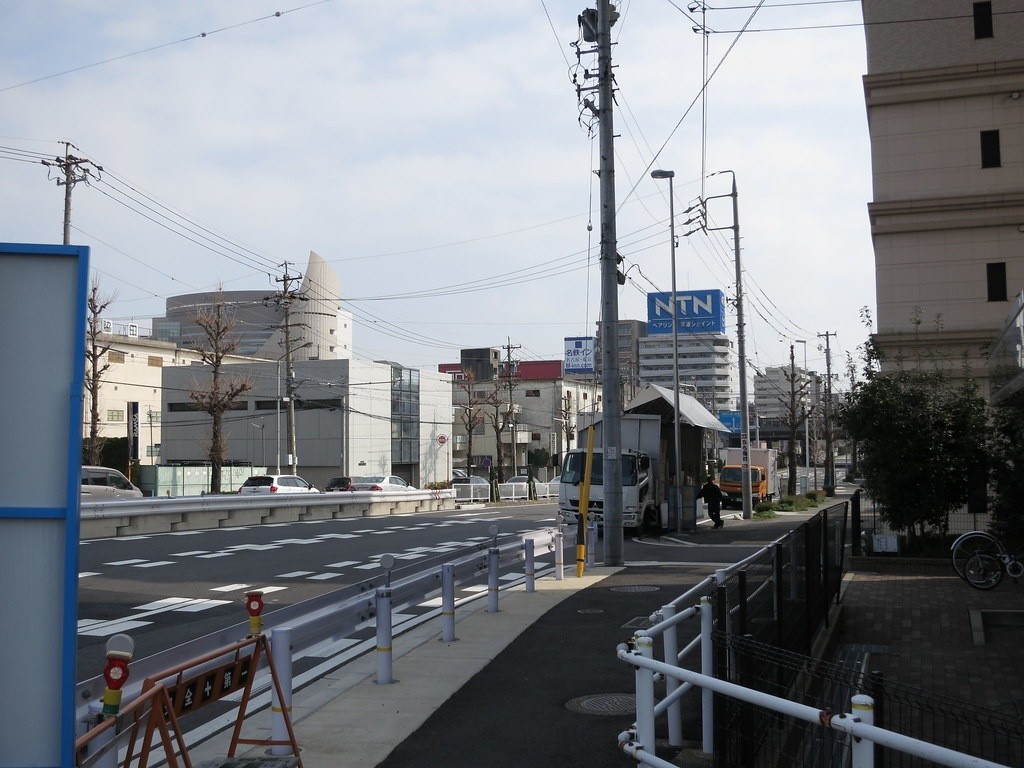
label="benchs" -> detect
[793,643,889,768]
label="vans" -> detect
[80,465,143,503]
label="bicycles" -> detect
[950,517,1024,590]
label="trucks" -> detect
[719,447,778,512]
[554,408,703,538]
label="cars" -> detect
[347,474,418,493]
[545,475,561,497]
[450,475,490,501]
[498,475,543,498]
[324,476,366,493]
[237,474,320,494]
[452,468,477,483]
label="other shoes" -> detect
[719,520,724,528]
[712,523,721,528]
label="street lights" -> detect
[276,342,313,475]
[650,169,685,538]
[795,338,809,496]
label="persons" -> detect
[696,477,724,529]
